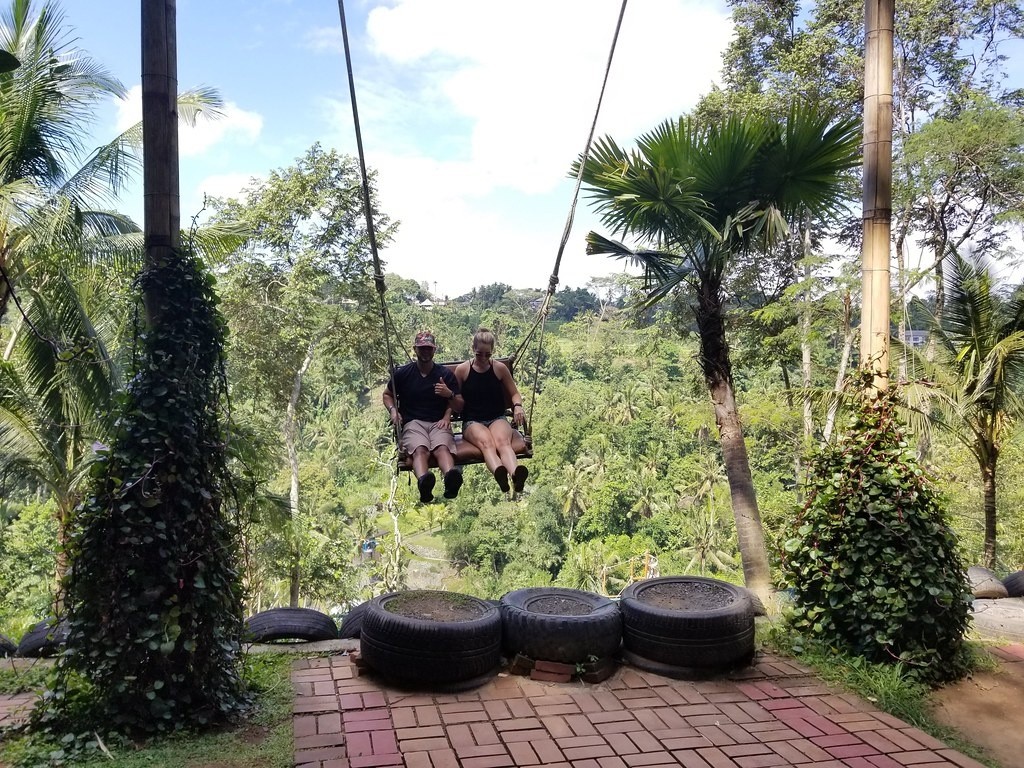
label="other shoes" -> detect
[512,465,529,492]
[444,468,463,498]
[418,471,435,503]
[494,465,510,493]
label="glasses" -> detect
[474,347,493,357]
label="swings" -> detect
[334,0,629,477]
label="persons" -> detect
[451,327,529,494]
[381,331,464,502]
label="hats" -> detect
[414,332,436,348]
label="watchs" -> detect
[514,402,522,408]
[449,392,455,400]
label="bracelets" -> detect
[388,405,397,412]
